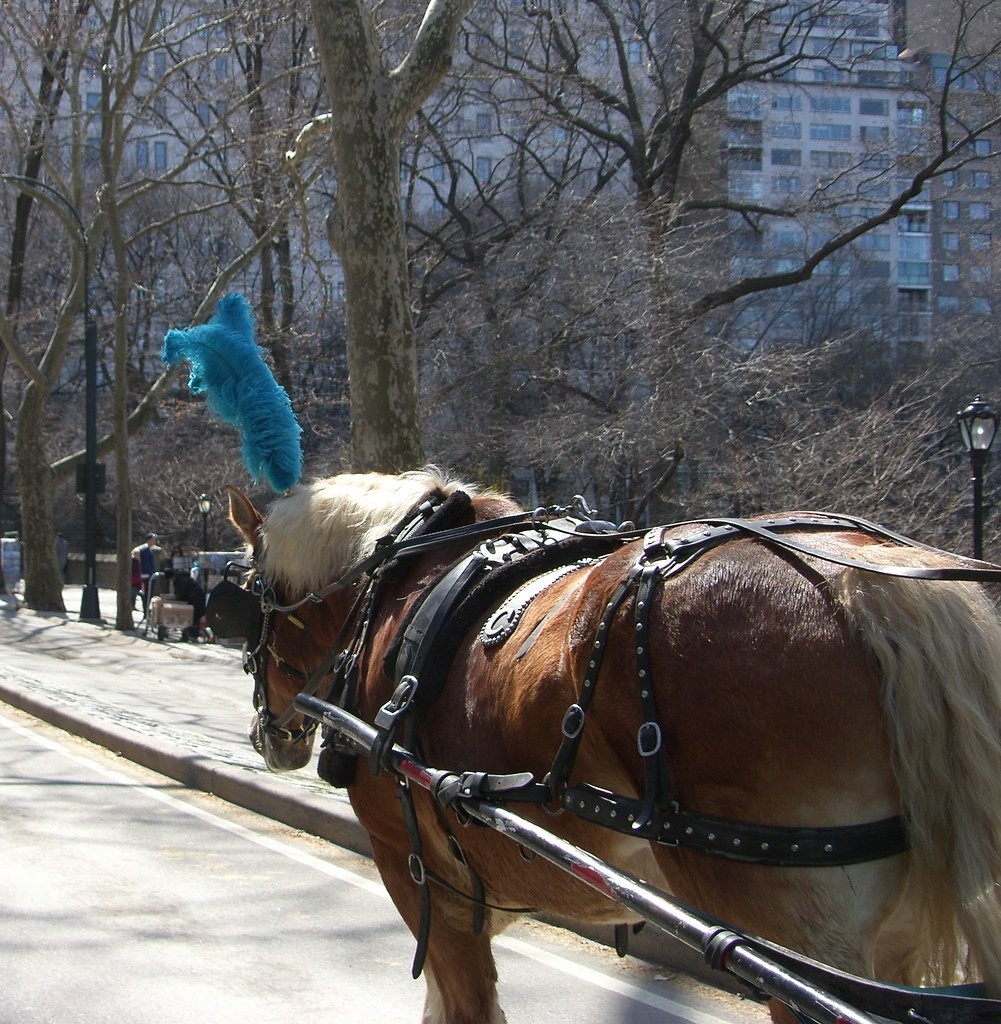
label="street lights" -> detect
[955,391,1000,560]
[198,492,211,591]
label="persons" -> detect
[0,531,207,644]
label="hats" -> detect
[164,568,175,579]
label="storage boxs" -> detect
[151,591,195,628]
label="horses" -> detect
[224,463,1001,1023]
